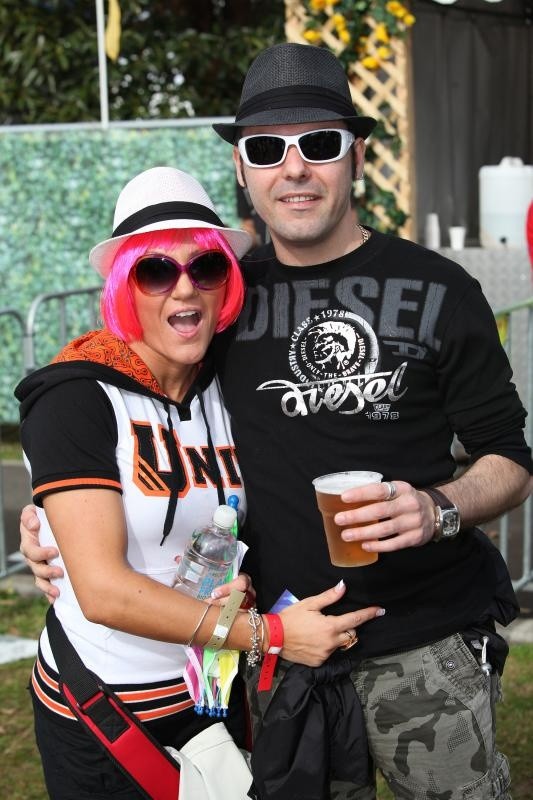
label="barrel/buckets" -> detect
[479,163,533,250]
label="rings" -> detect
[382,481,396,501]
[341,630,358,652]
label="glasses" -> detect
[236,128,357,168]
[129,248,232,296]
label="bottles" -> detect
[167,505,238,604]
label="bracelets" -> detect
[187,591,283,692]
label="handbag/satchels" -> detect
[156,722,256,800]
[227,645,380,800]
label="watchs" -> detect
[418,487,460,542]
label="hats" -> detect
[90,167,253,280]
[211,42,378,141]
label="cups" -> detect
[424,213,441,250]
[448,226,466,250]
[312,470,384,568]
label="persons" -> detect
[13,166,387,800]
[20,44,533,800]
